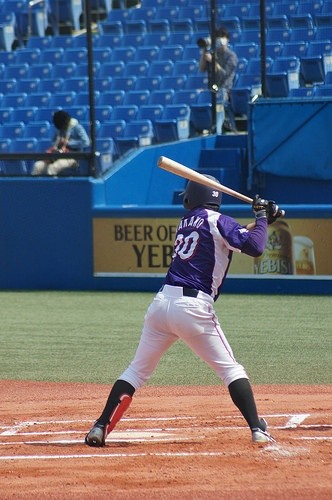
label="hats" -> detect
[53,110,68,128]
[215,26,227,37]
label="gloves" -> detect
[267,200,280,224]
[252,193,269,218]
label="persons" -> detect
[85,174,282,446]
[200,29,238,132]
[31,110,91,177]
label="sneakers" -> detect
[251,419,277,443]
[84,420,108,447]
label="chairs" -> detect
[0,0,331,176]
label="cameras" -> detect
[197,37,211,47]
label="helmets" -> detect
[178,173,223,211]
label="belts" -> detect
[159,285,199,297]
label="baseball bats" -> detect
[157,155,287,218]
